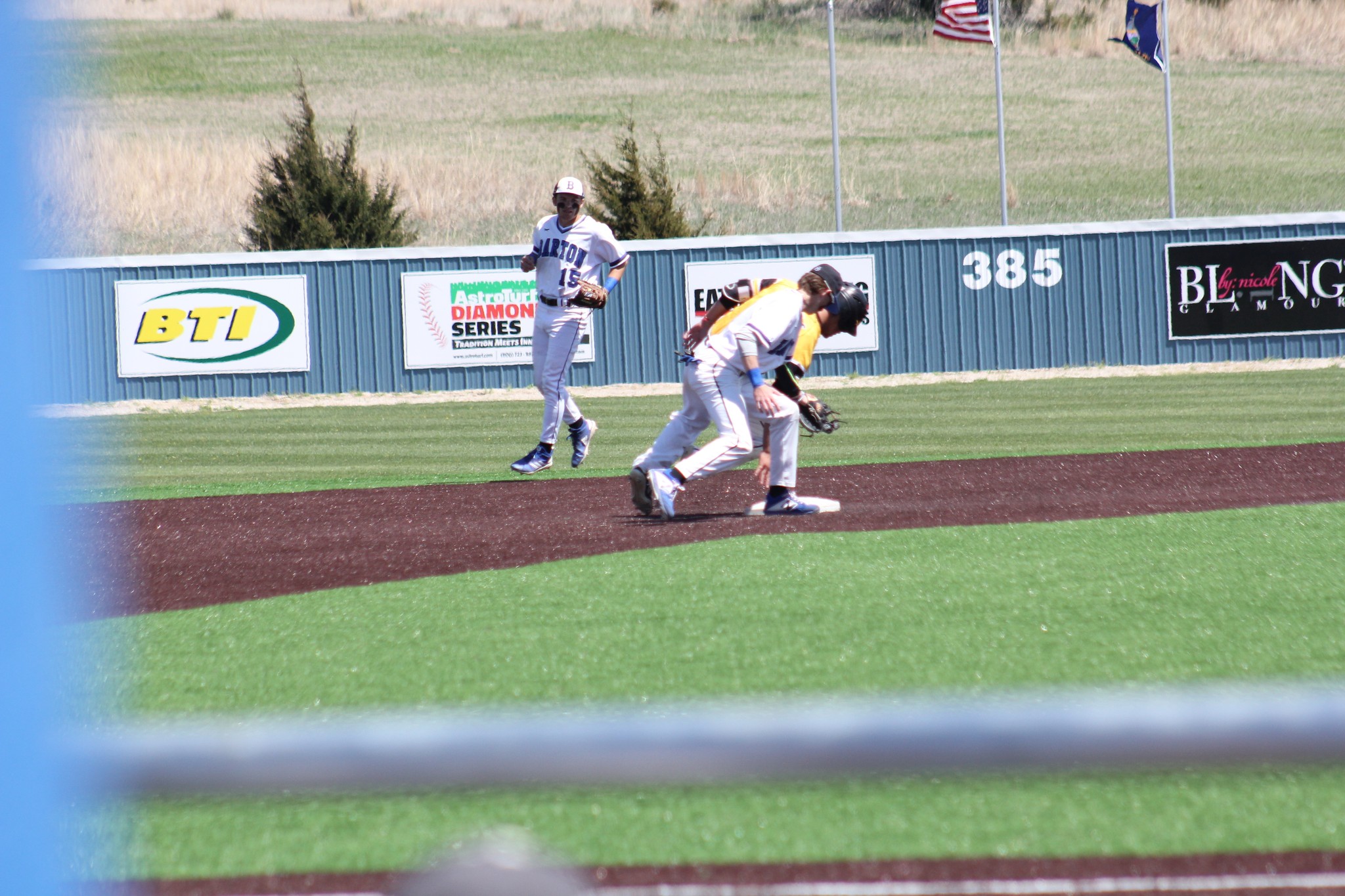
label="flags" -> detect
[932,0,996,48]
[1106,0,1165,74]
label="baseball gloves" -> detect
[799,392,840,434]
[568,279,608,309]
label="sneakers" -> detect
[629,467,653,516]
[566,415,596,468]
[763,491,820,515]
[647,469,680,518]
[511,444,555,474]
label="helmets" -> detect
[839,281,867,337]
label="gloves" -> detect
[674,349,703,366]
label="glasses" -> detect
[824,280,835,306]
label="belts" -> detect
[538,294,574,307]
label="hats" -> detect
[810,264,843,308]
[553,177,585,198]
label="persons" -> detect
[646,264,842,518]
[629,280,869,516]
[511,177,630,474]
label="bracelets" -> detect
[745,368,764,388]
